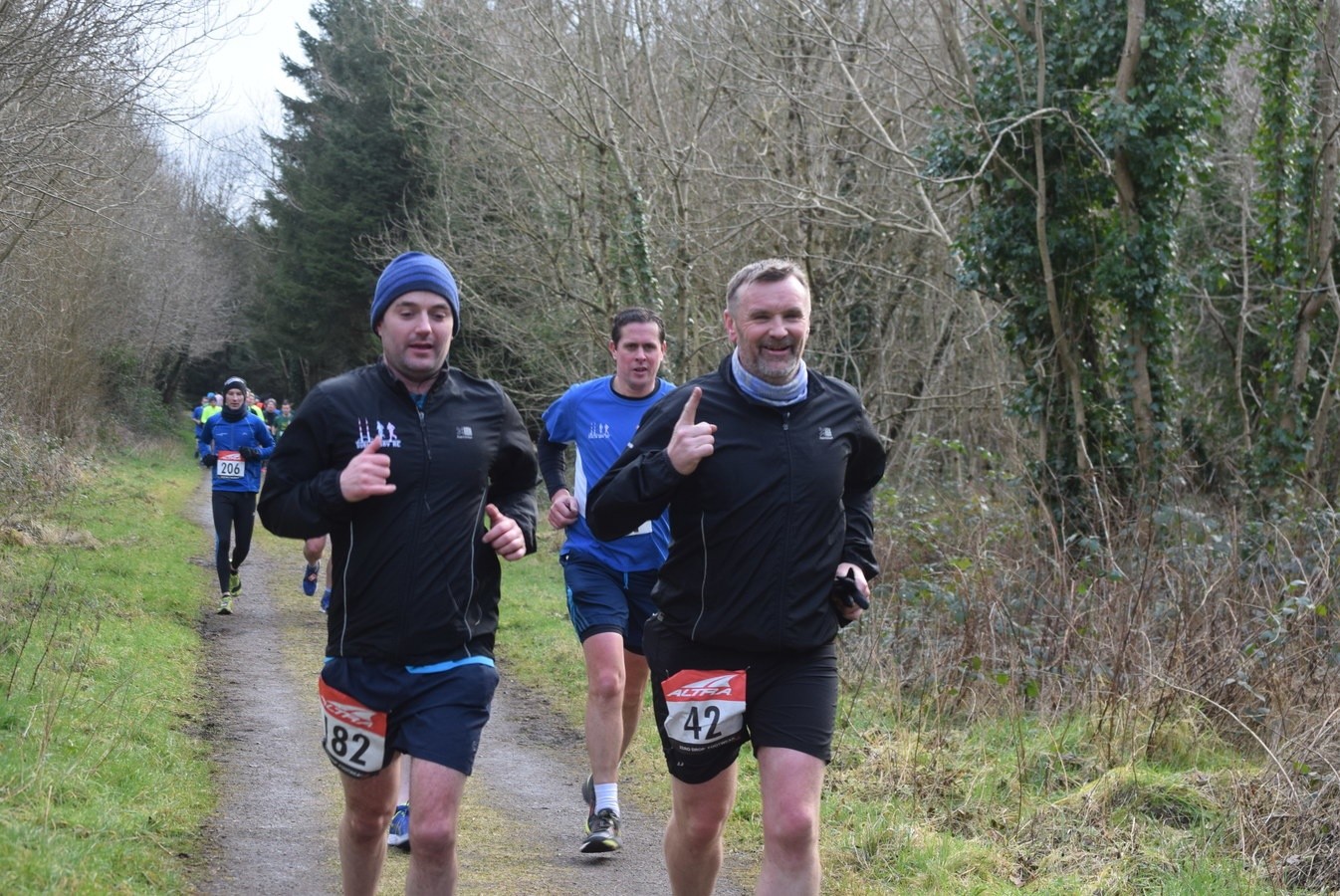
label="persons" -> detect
[386,753,411,852]
[198,376,276,614]
[303,534,333,613]
[535,306,679,853]
[258,251,539,896]
[192,387,297,472]
[585,254,887,896]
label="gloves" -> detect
[829,567,870,628]
[203,454,218,466]
[239,446,258,460]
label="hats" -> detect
[223,375,247,404]
[371,251,459,338]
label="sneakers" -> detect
[302,560,319,596]
[216,596,233,615]
[386,803,411,852]
[580,808,619,853]
[228,559,242,597]
[582,777,595,835]
[320,589,331,612]
